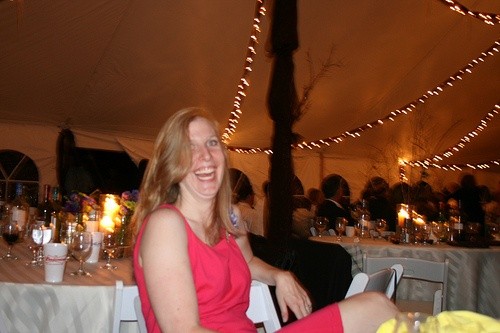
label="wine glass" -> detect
[0,192,111,279]
[313,216,496,246]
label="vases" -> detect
[116,214,132,247]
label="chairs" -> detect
[112,280,139,333]
[135,281,281,333]
[361,255,449,315]
[344,264,404,301]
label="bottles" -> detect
[400,199,464,244]
[357,199,370,237]
[10,184,28,239]
[39,183,53,224]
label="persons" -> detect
[316,173,359,236]
[129,107,414,333]
[234,183,266,238]
[226,164,500,247]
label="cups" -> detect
[85,232,101,264]
[42,243,70,282]
[345,225,355,237]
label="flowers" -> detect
[120,189,141,215]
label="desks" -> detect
[310,235,499,317]
[0,227,139,333]
